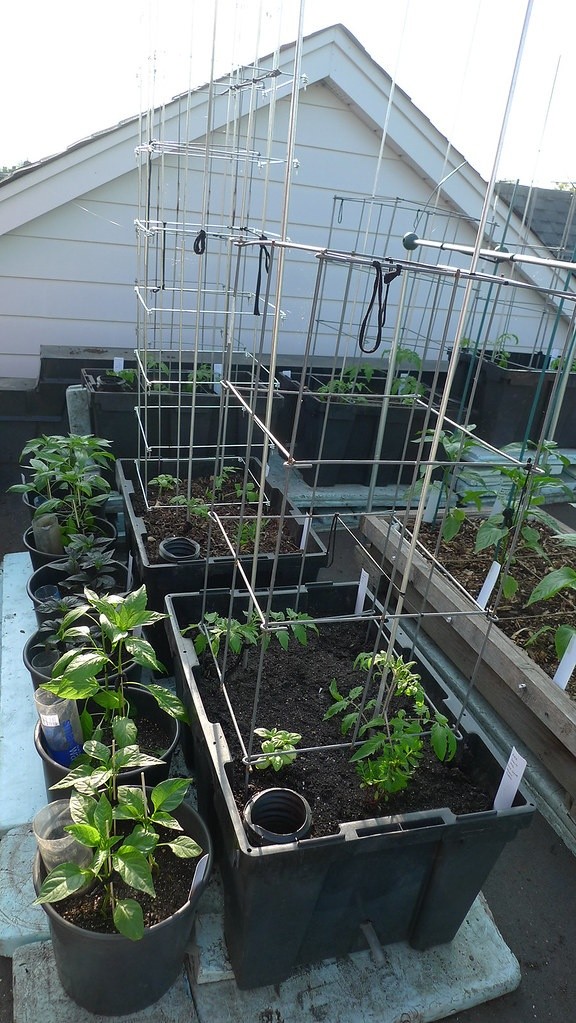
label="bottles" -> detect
[33,686,87,767]
[32,513,62,555]
[31,650,61,676]
[35,585,60,602]
[34,496,52,507]
[33,800,97,896]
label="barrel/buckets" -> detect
[242,786,312,847]
[156,536,201,561]
[97,375,127,392]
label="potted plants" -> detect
[2,331,575,1020]
